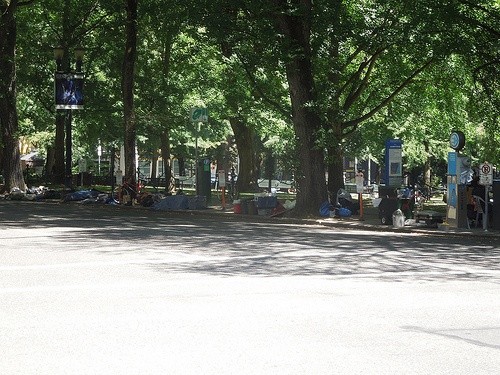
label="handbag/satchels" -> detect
[473,197,484,213]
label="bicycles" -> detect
[112,175,155,207]
[215,174,240,202]
